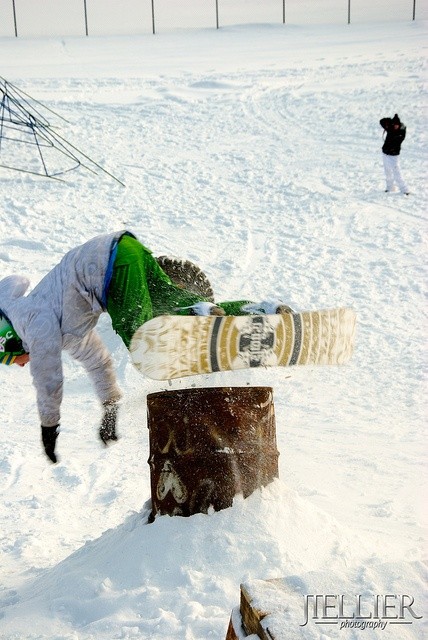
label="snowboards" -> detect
[130,306,357,381]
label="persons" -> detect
[379,113,413,196]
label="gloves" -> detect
[100,403,119,445]
[40,422,60,463]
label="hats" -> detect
[0,355,17,366]
[391,114,400,124]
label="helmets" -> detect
[0,311,25,365]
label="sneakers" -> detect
[385,190,388,192]
[176,302,225,315]
[240,298,295,314]
[406,193,409,195]
